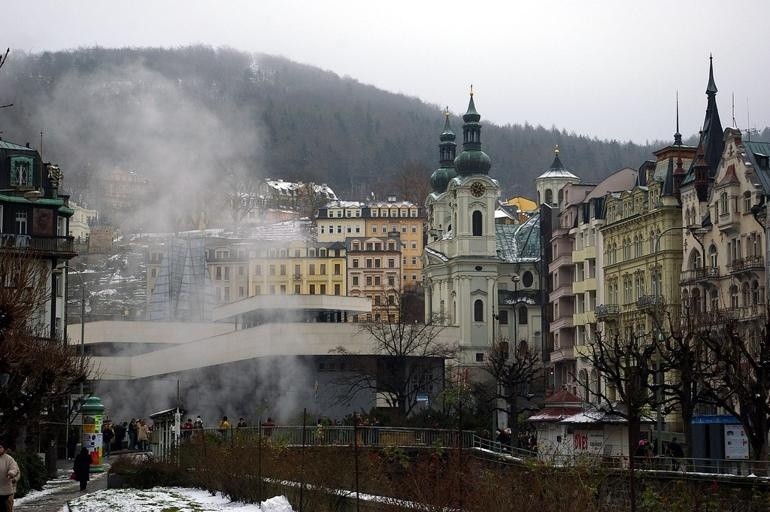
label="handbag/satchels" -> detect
[68,471,76,480]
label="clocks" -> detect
[468,180,487,199]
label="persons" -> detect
[0,444,21,512]
[193,415,205,427]
[170,421,180,430]
[353,412,380,445]
[237,418,247,427]
[633,435,685,470]
[184,419,195,428]
[494,429,510,452]
[317,417,322,425]
[260,418,277,435]
[503,428,514,453]
[219,415,230,428]
[72,447,92,491]
[101,416,153,459]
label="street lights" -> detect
[654,224,702,471]
[56,264,85,459]
[492,272,518,451]
